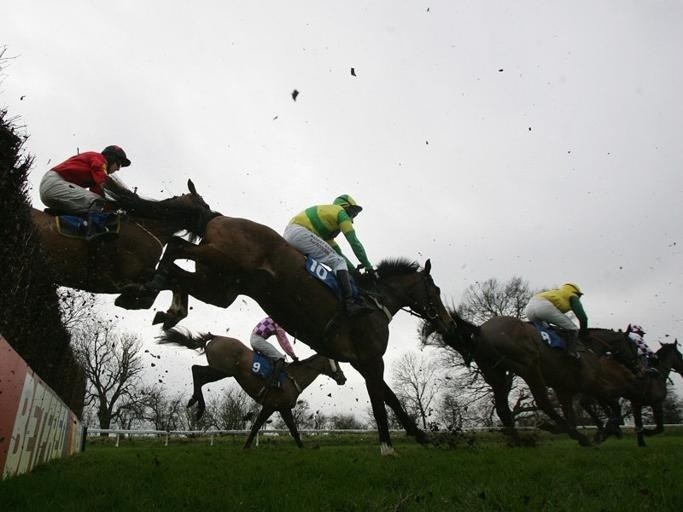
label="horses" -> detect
[575,338,682,439]
[418,293,648,446]
[152,323,347,451]
[101,170,458,456]
[26,178,213,310]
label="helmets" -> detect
[560,283,584,295]
[633,325,647,334]
[333,195,362,212]
[101,146,131,167]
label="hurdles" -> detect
[2,112,88,478]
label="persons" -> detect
[628,325,658,376]
[283,195,374,316]
[250,318,298,389]
[40,145,130,245]
[526,283,588,360]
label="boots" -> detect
[336,270,370,318]
[271,358,285,389]
[565,329,582,361]
[86,213,119,245]
